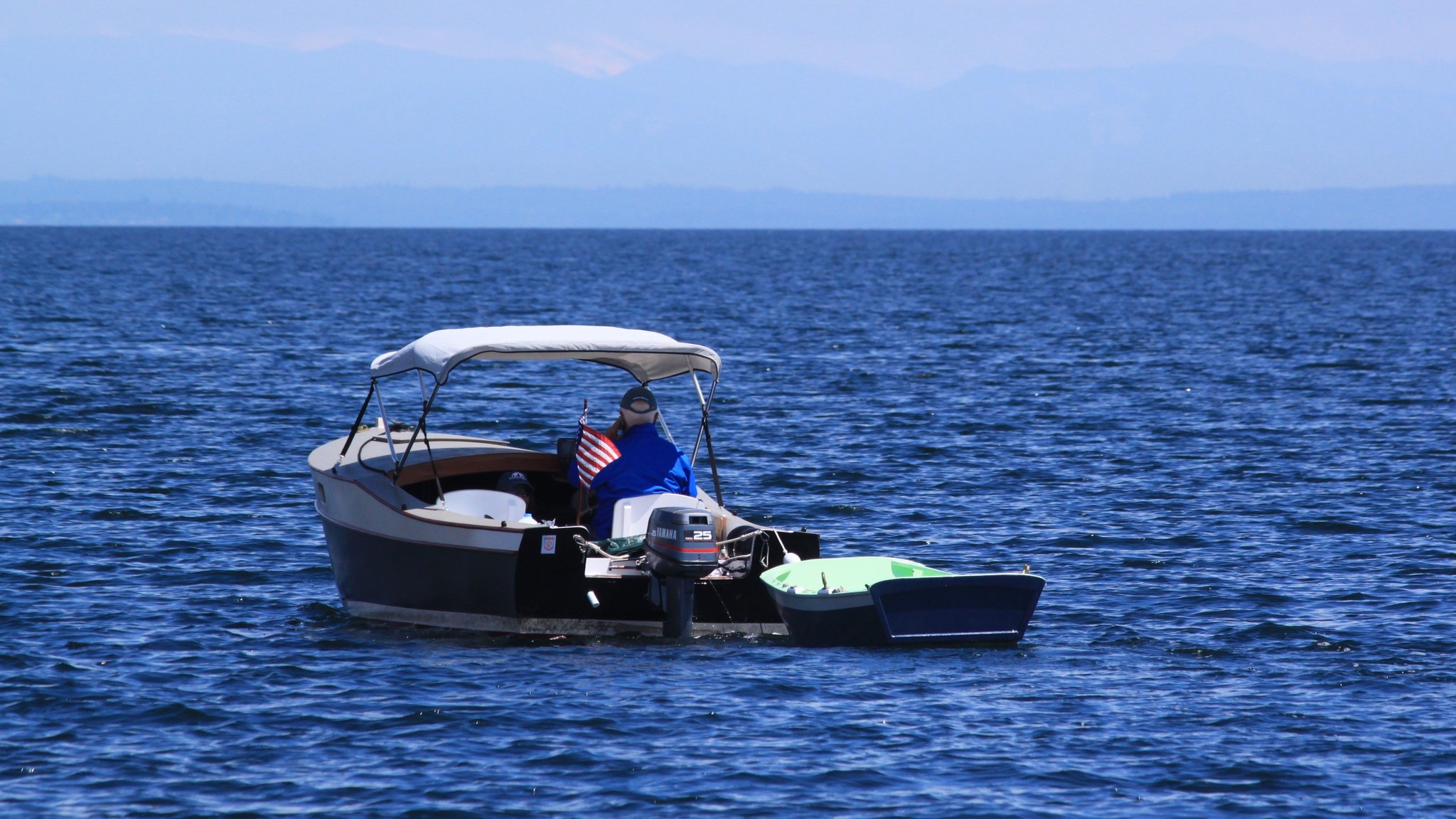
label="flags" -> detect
[569,415,621,493]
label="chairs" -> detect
[436,489,528,525]
[611,493,705,539]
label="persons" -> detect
[569,385,697,543]
[498,472,536,514]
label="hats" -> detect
[620,387,657,413]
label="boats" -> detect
[307,326,823,640]
[758,555,1047,651]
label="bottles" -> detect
[518,513,538,524]
